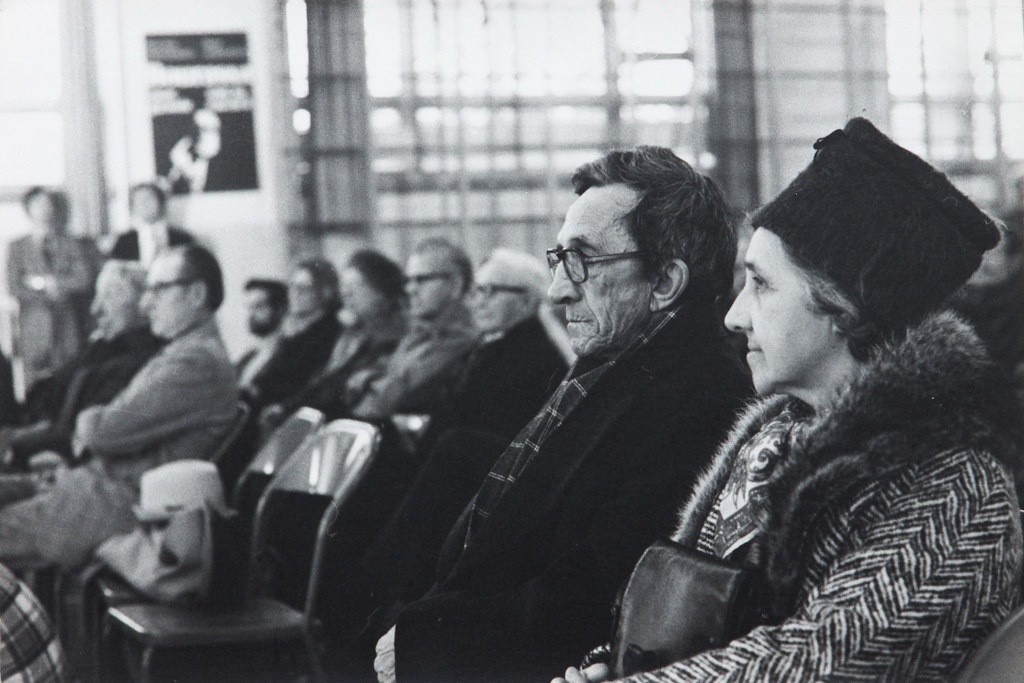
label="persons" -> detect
[231,257,350,424]
[230,279,292,382]
[340,234,483,422]
[369,146,758,683]
[543,112,1024,680]
[258,249,411,430]
[380,243,571,462]
[0,258,171,472]
[0,241,242,571]
[92,183,225,313]
[3,186,125,398]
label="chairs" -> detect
[50,397,1024,682]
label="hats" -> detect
[754,118,1006,332]
[135,459,230,522]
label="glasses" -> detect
[404,272,447,286]
[469,283,527,298]
[139,280,192,295]
[547,248,648,284]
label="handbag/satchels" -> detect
[98,498,235,609]
[611,537,753,679]
